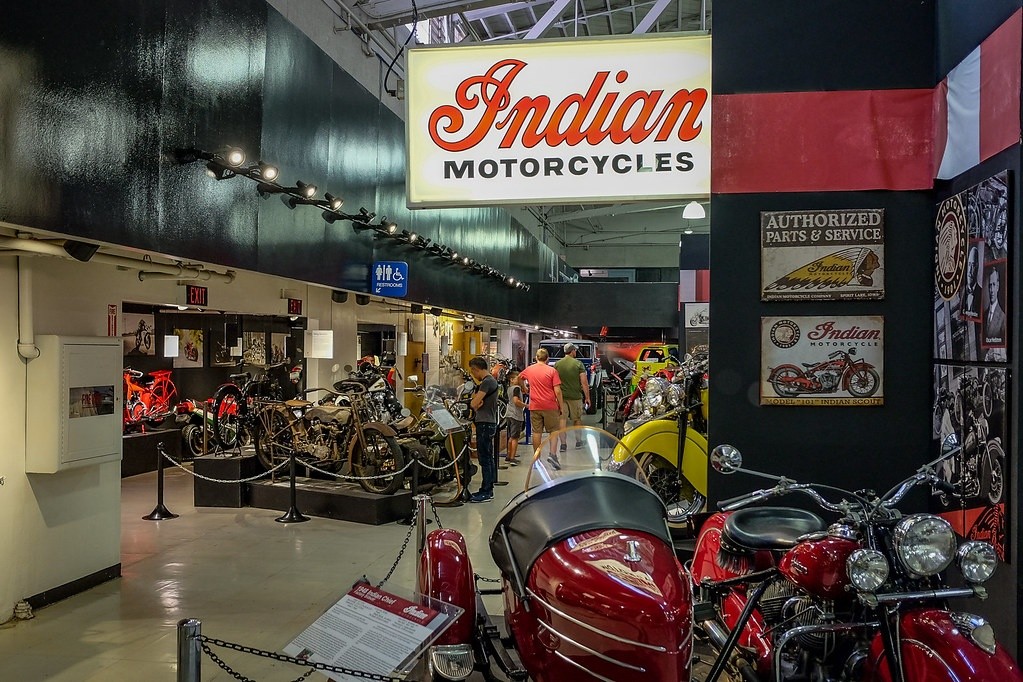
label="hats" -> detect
[564,342,579,353]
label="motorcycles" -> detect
[768,347,880,399]
[938,376,1005,506]
[174,395,253,457]
[123,365,177,429]
[184,342,198,361]
[251,375,404,493]
[421,434,1023,682]
[601,370,628,399]
[690,308,709,326]
[135,324,151,349]
[320,352,519,486]
[609,345,709,529]
[215,342,234,363]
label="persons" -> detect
[513,347,563,470]
[983,267,1006,343]
[961,247,982,319]
[468,355,499,503]
[553,341,592,451]
[505,372,530,465]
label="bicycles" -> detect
[961,182,1007,249]
[211,360,286,456]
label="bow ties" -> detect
[967,289,976,296]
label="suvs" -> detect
[535,338,601,413]
[635,344,680,377]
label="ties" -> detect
[988,307,992,330]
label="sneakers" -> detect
[547,453,561,470]
[576,440,587,448]
[533,460,547,468]
[504,458,521,465]
[559,444,567,451]
[470,492,494,503]
